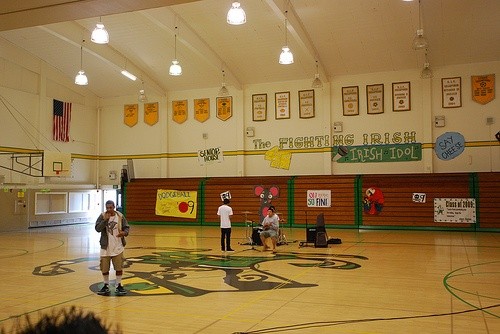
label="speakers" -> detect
[306,227,316,242]
[252,228,264,245]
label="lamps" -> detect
[422,50,433,79]
[218,71,228,93]
[311,57,323,89]
[226,0,246,25]
[91,16,109,44]
[279,11,295,64]
[138,81,147,102]
[414,0,428,50]
[169,27,182,76]
[75,42,88,85]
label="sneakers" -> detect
[97,284,110,295]
[115,283,128,294]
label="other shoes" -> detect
[221,247,233,251]
[272,249,277,253]
[263,245,267,251]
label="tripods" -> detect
[243,216,262,253]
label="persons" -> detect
[95,200,130,295]
[216,199,235,252]
[269,206,280,239]
[259,209,279,253]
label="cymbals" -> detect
[275,212,283,215]
[241,212,254,214]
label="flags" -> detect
[52,99,73,142]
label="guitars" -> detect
[264,220,274,230]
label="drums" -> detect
[252,226,263,244]
